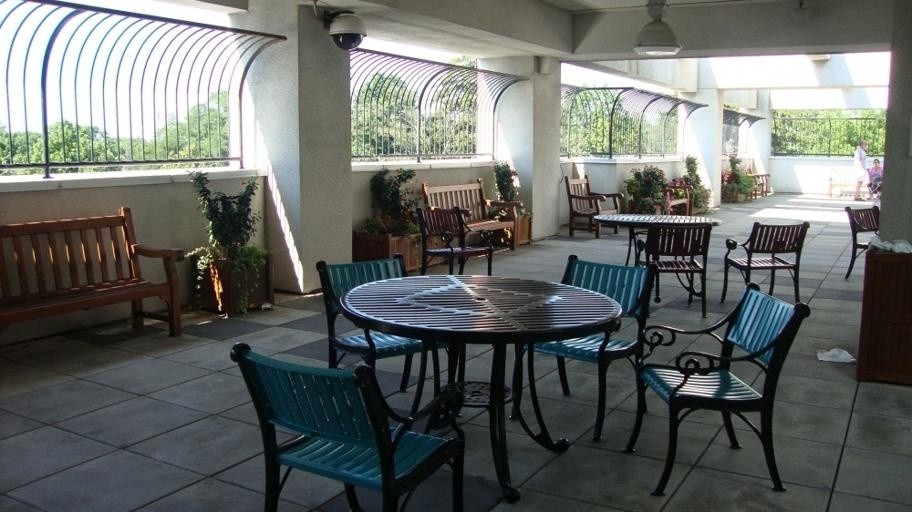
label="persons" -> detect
[853,141,868,201]
[869,158,883,195]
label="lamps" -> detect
[323,7,367,52]
[629,0,684,58]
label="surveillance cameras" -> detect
[328,13,368,52]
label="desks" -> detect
[594,213,722,296]
[339,272,625,504]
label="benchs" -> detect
[487,161,531,247]
[1,206,182,337]
[418,177,523,251]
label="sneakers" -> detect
[854,196,866,202]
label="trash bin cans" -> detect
[856,242,912,386]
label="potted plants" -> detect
[682,155,710,214]
[354,162,449,271]
[624,164,669,215]
[721,156,756,202]
[178,167,277,317]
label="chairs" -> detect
[312,253,449,413]
[229,339,465,511]
[722,220,811,307]
[418,205,493,280]
[500,252,658,444]
[747,163,770,197]
[636,218,709,320]
[844,204,879,281]
[619,281,815,495]
[564,175,625,239]
[662,183,693,216]
[741,162,760,200]
